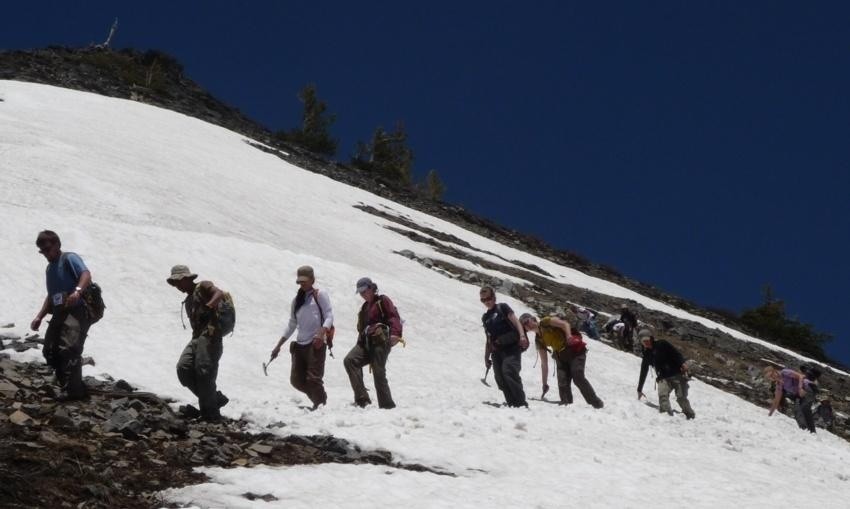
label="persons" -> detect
[478,286,530,410]
[31,230,92,403]
[270,265,333,411]
[166,264,230,424]
[799,365,821,386]
[518,313,604,409]
[571,304,638,352]
[636,329,696,420]
[344,277,403,410]
[762,365,817,433]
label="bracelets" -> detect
[519,334,526,338]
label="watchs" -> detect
[74,286,84,295]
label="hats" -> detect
[518,313,532,324]
[639,330,651,343]
[480,287,493,301]
[167,266,197,287]
[295,266,314,283]
[356,278,371,292]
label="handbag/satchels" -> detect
[206,293,234,336]
[680,359,703,380]
[494,329,529,353]
[565,328,586,353]
[80,283,105,322]
[362,324,389,346]
[800,364,820,380]
[322,325,335,349]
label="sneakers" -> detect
[196,395,229,424]
[352,398,371,407]
